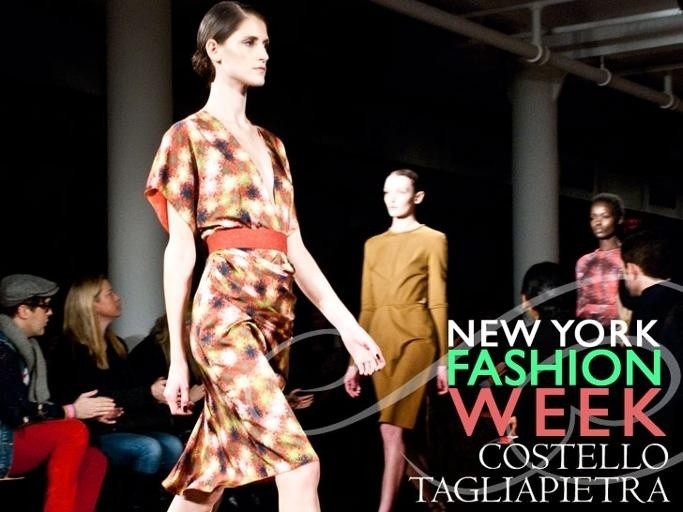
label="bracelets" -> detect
[65,403,77,419]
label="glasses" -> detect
[22,301,52,310]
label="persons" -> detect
[129,306,207,438]
[1,273,126,512]
[145,0,388,512]
[47,274,185,512]
[617,229,683,353]
[341,167,452,511]
[505,262,602,384]
[575,191,632,340]
[284,387,314,411]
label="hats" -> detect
[1,275,59,308]
